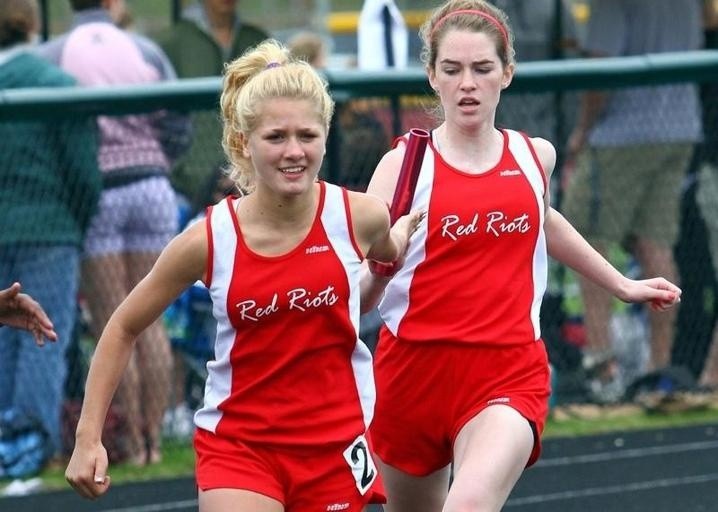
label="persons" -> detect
[360,0,681,512]
[492,0,718,405]
[66,44,427,512]
[0,0,325,495]
